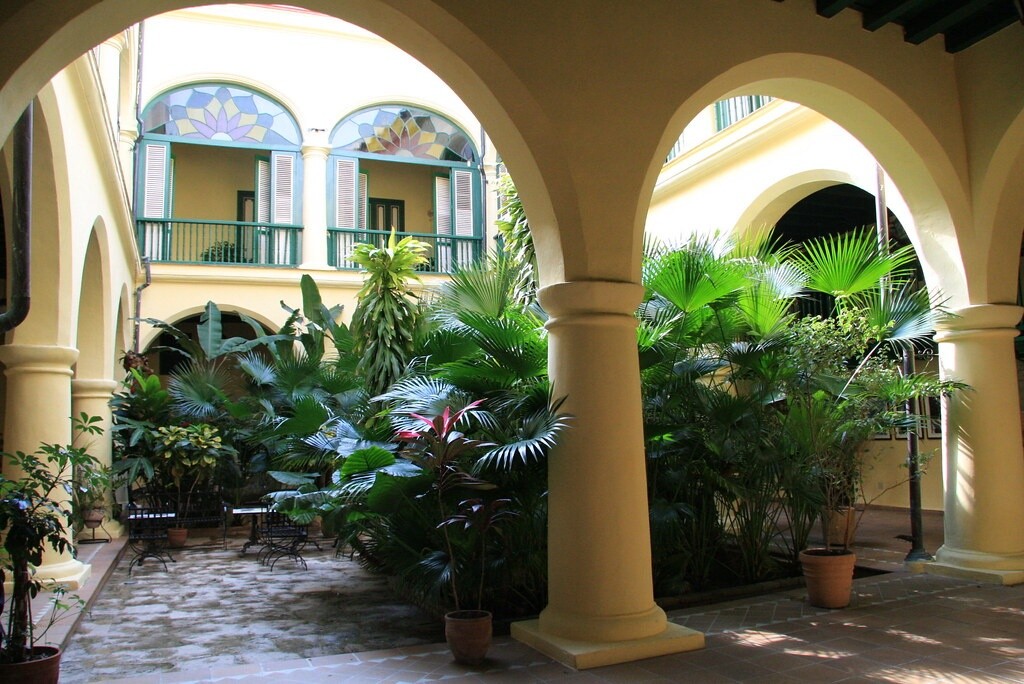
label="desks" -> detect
[129,513,175,539]
[232,507,277,546]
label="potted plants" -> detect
[76,463,123,529]
[736,314,938,610]
[0,405,128,684]
[397,395,526,668]
[151,424,222,549]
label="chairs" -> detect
[126,518,176,576]
[257,523,312,571]
